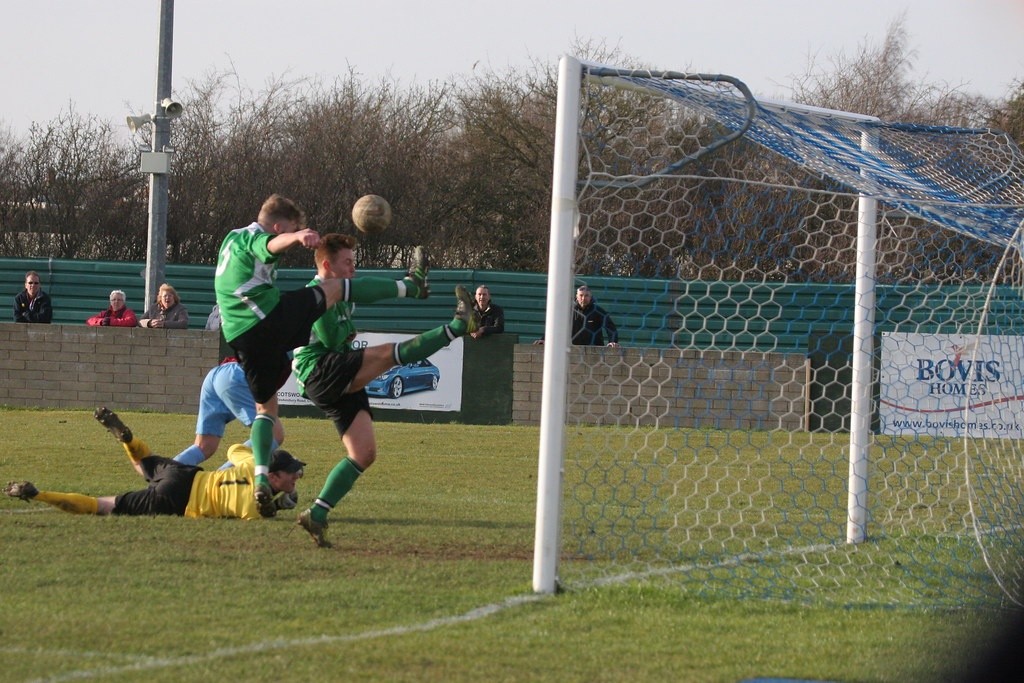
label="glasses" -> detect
[25,281,39,285]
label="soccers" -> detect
[350,192,392,237]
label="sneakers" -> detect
[296,507,332,549]
[455,284,479,334]
[253,485,278,519]
[405,245,430,299]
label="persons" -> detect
[533,285,621,348]
[3,406,306,520]
[470,285,504,339]
[205,304,220,331]
[13,271,52,323]
[86,289,137,326]
[214,193,430,517]
[292,234,480,548]
[138,283,188,329]
[173,355,285,470]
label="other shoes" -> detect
[92,407,133,444]
[1,479,39,503]
